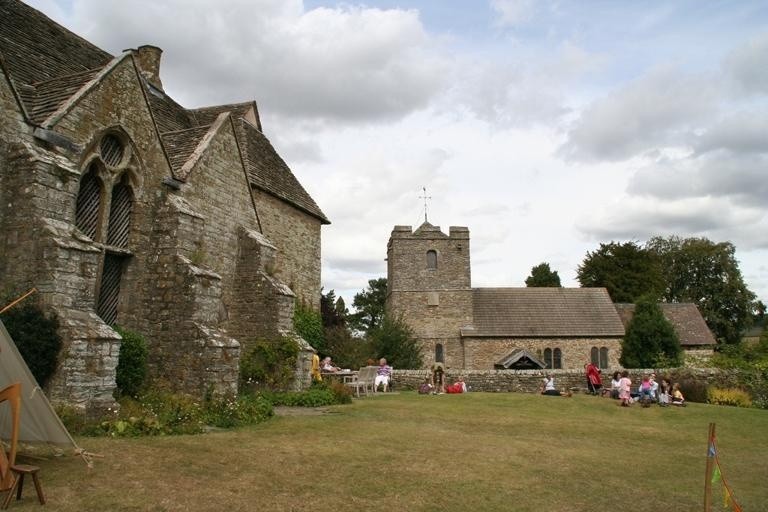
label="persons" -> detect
[454,375,468,392]
[429,361,446,395]
[366,358,375,368]
[312,349,342,383]
[608,369,685,408]
[536,372,573,397]
[373,358,393,393]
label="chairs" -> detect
[0,382,45,512]
[344,366,393,398]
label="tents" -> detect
[0,318,80,460]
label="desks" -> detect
[321,371,355,383]
